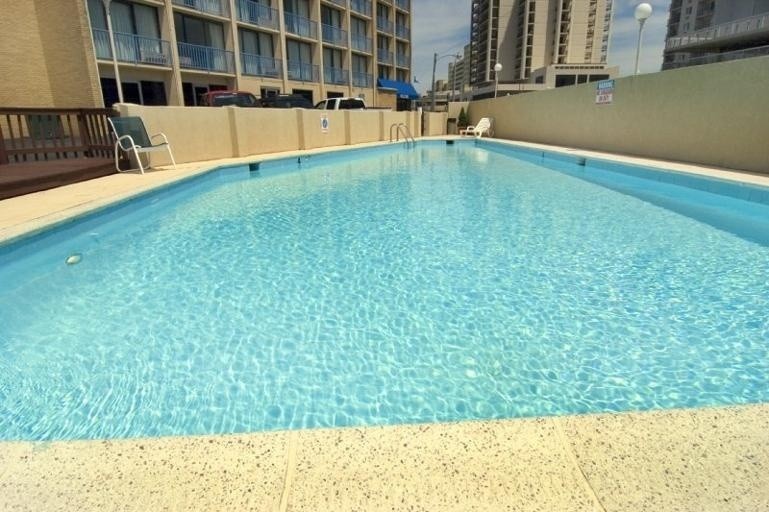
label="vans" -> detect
[200,91,314,109]
[315,97,365,109]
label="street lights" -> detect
[430,53,462,112]
[494,63,503,99]
[634,3,652,75]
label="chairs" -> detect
[459,116,495,139]
[105,115,175,174]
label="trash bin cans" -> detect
[447,118,456,134]
